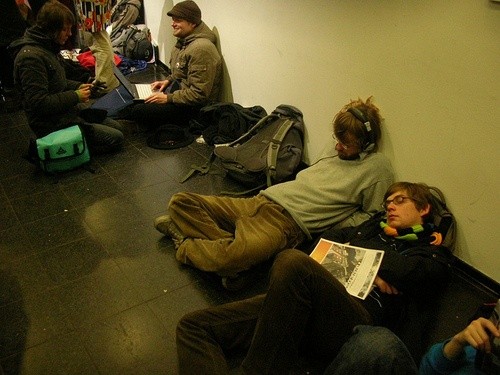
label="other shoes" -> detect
[154,215,187,247]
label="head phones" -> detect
[347,107,375,152]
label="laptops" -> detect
[110,60,163,102]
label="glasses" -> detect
[381,195,424,209]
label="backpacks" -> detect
[214,104,304,181]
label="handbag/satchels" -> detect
[117,24,153,61]
[36,126,90,173]
[205,105,267,146]
[108,0,141,36]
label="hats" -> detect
[167,0,202,25]
